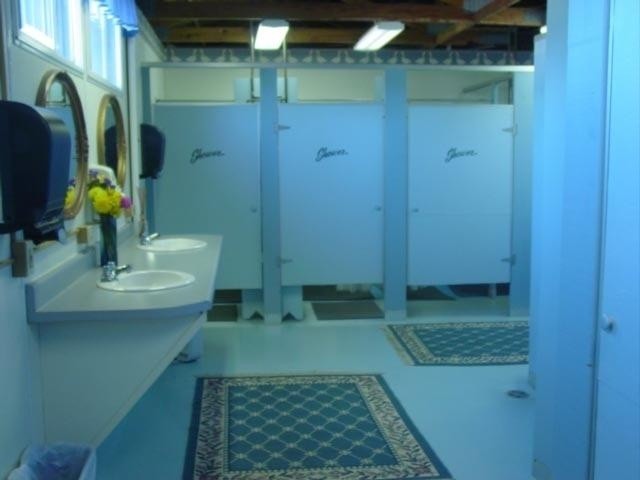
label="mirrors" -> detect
[97,94,126,191]
[36,69,90,220]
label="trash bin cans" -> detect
[172,329,202,363]
[8,443,96,480]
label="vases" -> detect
[98,215,118,265]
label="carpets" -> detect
[382,320,529,367]
[183,373,455,480]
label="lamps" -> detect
[352,20,406,51]
[253,19,292,51]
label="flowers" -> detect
[65,177,79,209]
[87,163,132,219]
